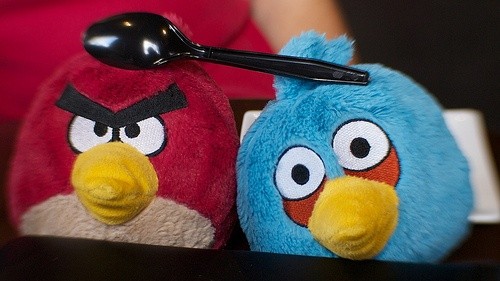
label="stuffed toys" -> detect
[234,29,474,266]
[4,14,241,250]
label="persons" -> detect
[0,0,362,125]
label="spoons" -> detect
[84,13,369,86]
